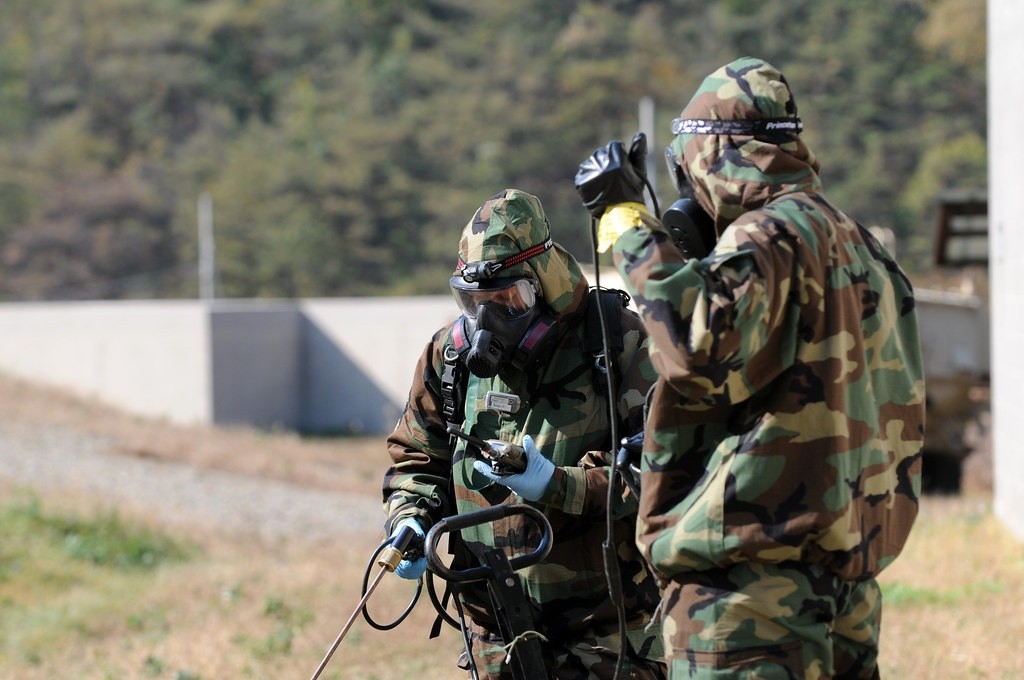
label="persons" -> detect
[383,189,666,680]
[573,55,925,680]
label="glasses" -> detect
[449,275,538,322]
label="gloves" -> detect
[474,434,556,501]
[385,517,428,580]
[573,131,649,218]
